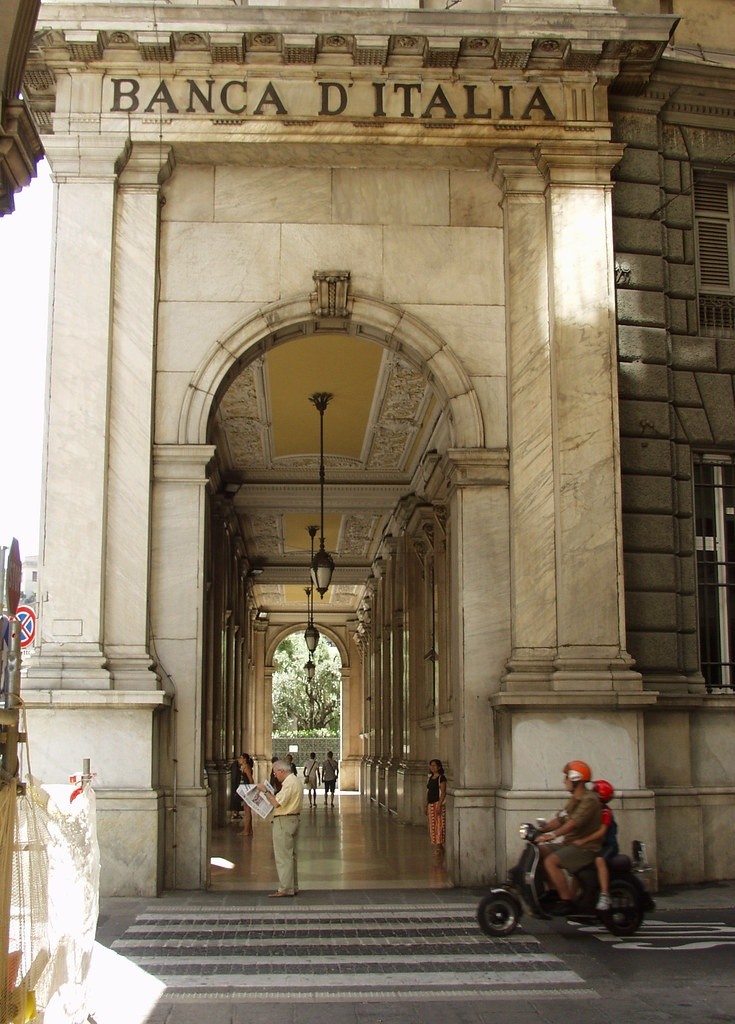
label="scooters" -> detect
[475,817,660,936]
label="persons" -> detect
[230,755,297,819]
[236,753,254,835]
[304,751,320,807]
[536,760,617,910]
[322,751,339,807]
[427,759,447,857]
[256,760,303,897]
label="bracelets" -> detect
[264,790,273,794]
[543,831,556,838]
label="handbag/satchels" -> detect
[304,775,309,785]
[438,775,446,805]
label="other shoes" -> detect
[551,900,578,915]
[538,891,558,903]
[268,891,297,897]
[596,894,612,910]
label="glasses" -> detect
[274,771,281,776]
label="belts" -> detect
[274,813,296,817]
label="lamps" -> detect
[303,391,335,683]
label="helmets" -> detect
[589,780,614,804]
[563,760,590,781]
